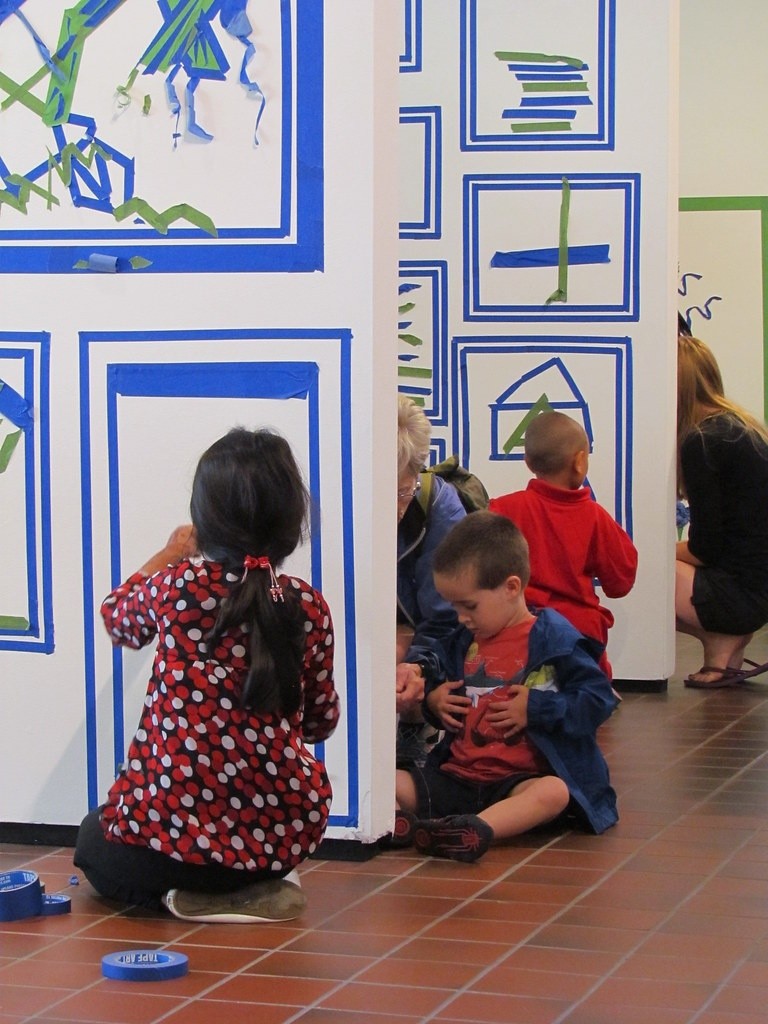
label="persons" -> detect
[396,511,619,861]
[396,394,468,760]
[489,411,638,685]
[677,335,768,689]
[73,426,340,924]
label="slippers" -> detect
[683,656,768,688]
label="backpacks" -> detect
[409,455,490,540]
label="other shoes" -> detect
[162,878,307,923]
[393,807,419,847]
[410,815,495,862]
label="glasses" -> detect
[398,478,420,502]
